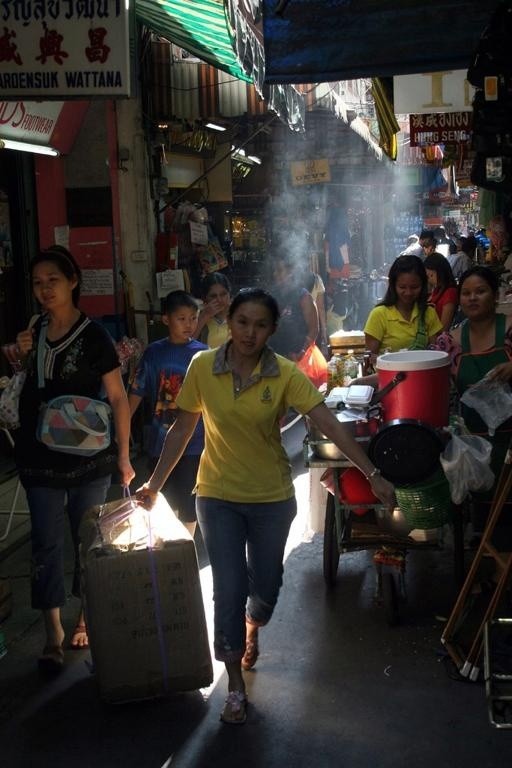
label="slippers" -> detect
[241,639,258,670]
[70,626,89,650]
[37,646,65,674]
[219,690,248,724]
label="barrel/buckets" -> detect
[320,466,382,516]
[371,350,450,446]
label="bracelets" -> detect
[304,334,314,343]
[367,468,380,482]
[322,326,327,329]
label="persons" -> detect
[122,291,215,545]
[135,289,394,724]
[366,220,511,413]
[260,255,319,361]
[289,250,330,342]
[193,272,237,349]
[0,244,134,677]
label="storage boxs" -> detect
[81,489,214,707]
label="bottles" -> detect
[326,350,374,391]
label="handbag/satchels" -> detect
[1,370,27,431]
[155,229,234,299]
[36,395,113,457]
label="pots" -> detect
[302,404,381,461]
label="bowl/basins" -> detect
[376,506,416,537]
